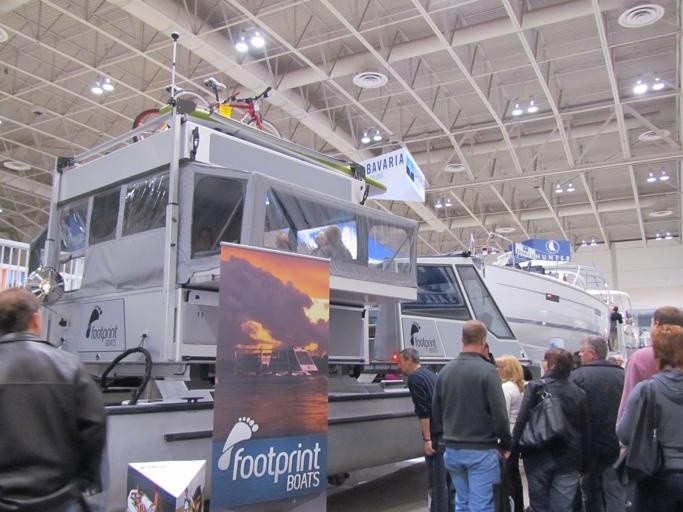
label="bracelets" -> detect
[423,438,431,442]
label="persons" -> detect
[399,348,457,511]
[497,305,682,511]
[131,484,201,512]
[275,225,353,263]
[431,320,512,511]
[0,288,108,512]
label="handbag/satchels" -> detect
[519,399,564,447]
[614,395,662,485]
[493,485,523,512]
